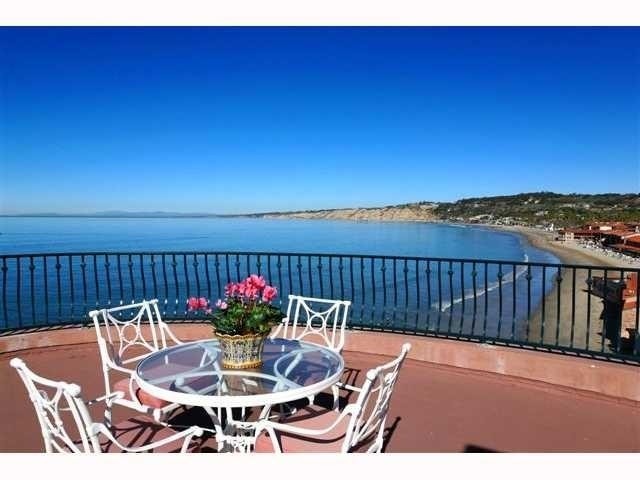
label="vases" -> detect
[218,331,266,368]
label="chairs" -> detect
[269,294,353,415]
[251,344,411,451]
[88,298,223,425]
[9,356,205,453]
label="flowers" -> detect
[185,273,285,338]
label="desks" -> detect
[134,336,343,453]
